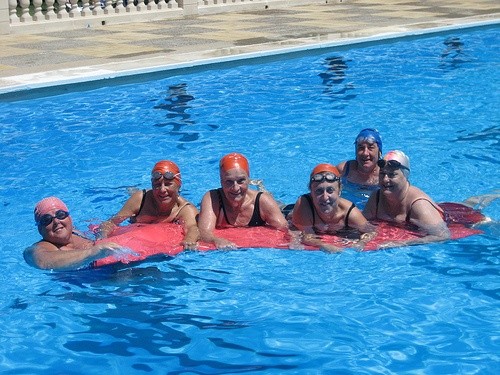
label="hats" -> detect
[353,128,382,153]
[382,150,410,181]
[310,164,341,177]
[151,160,181,188]
[220,153,249,176]
[34,196,68,223]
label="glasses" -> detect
[309,174,340,188]
[376,160,410,171]
[39,210,69,226]
[356,136,378,146]
[152,172,181,181]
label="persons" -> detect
[294,163,375,254]
[101,159,199,251]
[23,196,121,271]
[336,128,383,189]
[361,150,449,245]
[196,153,304,254]
